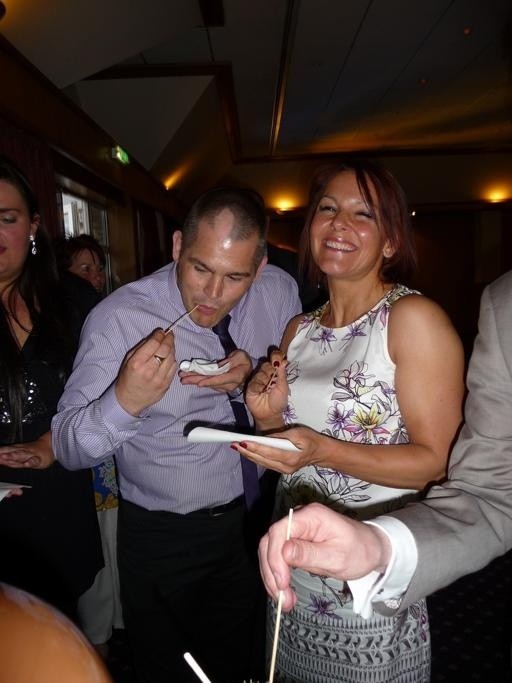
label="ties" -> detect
[211,313,260,510]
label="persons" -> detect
[1,161,107,605]
[229,153,466,683]
[256,270,511,613]
[48,179,302,682]
[61,231,128,659]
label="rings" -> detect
[153,353,165,364]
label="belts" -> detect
[193,500,242,519]
